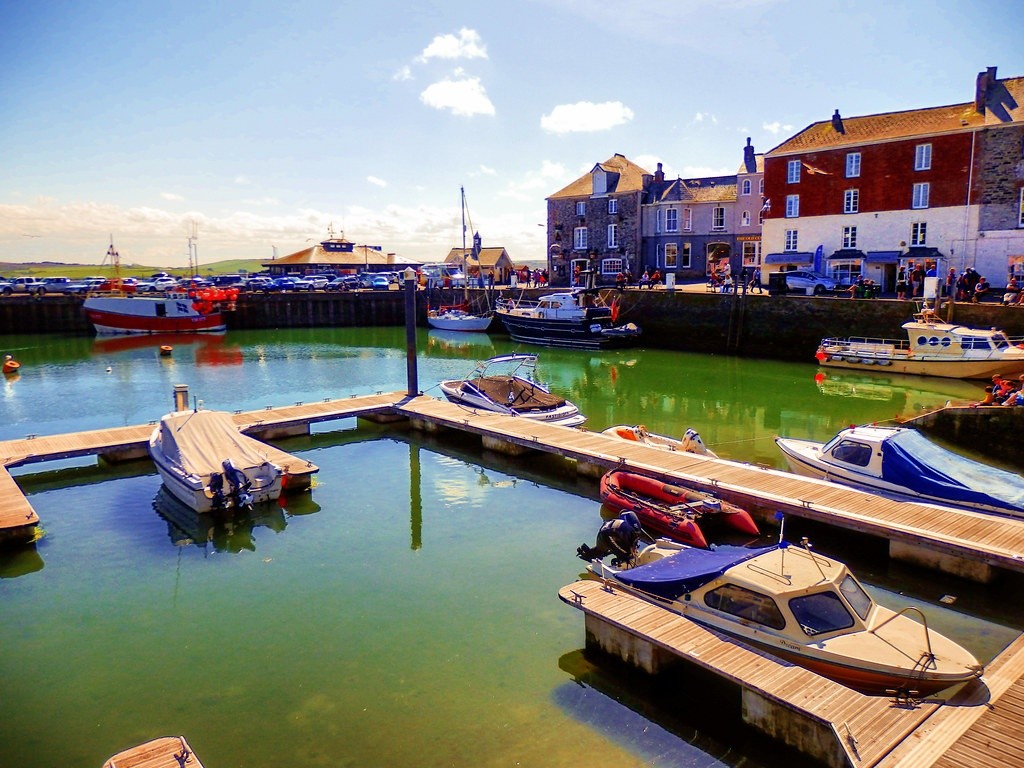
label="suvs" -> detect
[784,269,840,294]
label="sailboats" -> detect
[426,186,494,331]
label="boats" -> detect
[438,350,591,427]
[601,424,719,460]
[240,432,320,493]
[494,283,649,351]
[576,457,984,698]
[815,301,1024,382]
[90,332,226,354]
[152,483,216,546]
[599,466,762,551]
[83,295,228,335]
[148,395,283,515]
[772,421,1023,523]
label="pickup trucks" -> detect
[24,277,82,296]
[0,277,43,296]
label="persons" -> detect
[324,283,327,291]
[750,266,763,293]
[574,266,581,286]
[711,262,733,293]
[526,268,548,288]
[617,272,628,284]
[999,273,1024,306]
[945,266,990,302]
[897,263,937,300]
[970,373,1024,407]
[508,298,515,309]
[846,275,875,299]
[487,270,495,289]
[638,269,661,289]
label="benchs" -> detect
[993,288,1022,304]
[706,279,749,293]
[639,279,663,288]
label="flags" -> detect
[611,299,619,322]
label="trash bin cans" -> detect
[769,273,786,296]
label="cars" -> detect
[63,262,471,290]
[0,276,17,283]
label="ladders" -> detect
[726,275,748,354]
